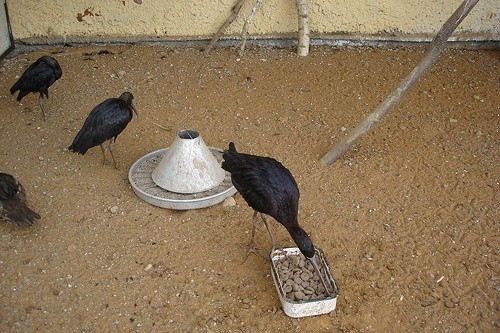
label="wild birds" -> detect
[68,92,139,170]
[221,142,315,266]
[9,55,62,121]
[0,173,41,228]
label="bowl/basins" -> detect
[269,245,339,318]
